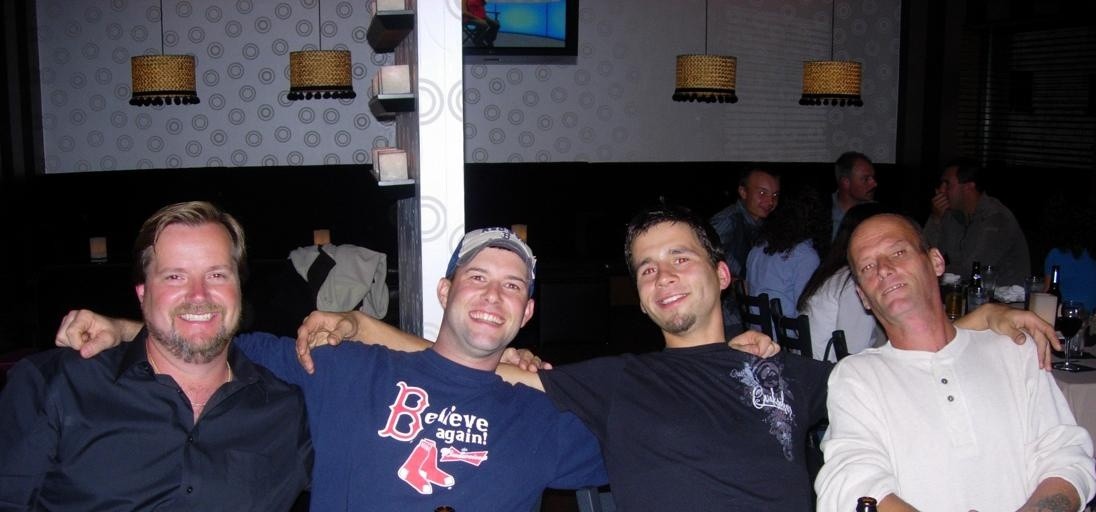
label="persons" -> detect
[0,201,555,512]
[52,223,790,512]
[708,149,1045,367]
[812,210,1095,512]
[293,202,1066,511]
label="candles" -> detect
[89,236,108,262]
[313,230,330,245]
[510,225,528,245]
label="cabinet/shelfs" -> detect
[357,7,416,192]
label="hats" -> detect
[445,225,537,296]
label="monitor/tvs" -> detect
[461,0,579,66]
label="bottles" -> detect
[970,261,986,310]
[1048,264,1064,330]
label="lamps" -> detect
[285,0,359,101]
[795,0,866,110]
[128,0,202,110]
[668,0,743,107]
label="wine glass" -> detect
[1054,300,1084,372]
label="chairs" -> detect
[731,278,775,350]
[572,485,616,512]
[769,292,815,360]
[823,324,858,365]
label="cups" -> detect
[1071,328,1085,358]
[944,283,966,322]
[856,496,878,511]
[1023,275,1046,312]
[1030,292,1058,330]
[983,267,1006,304]
